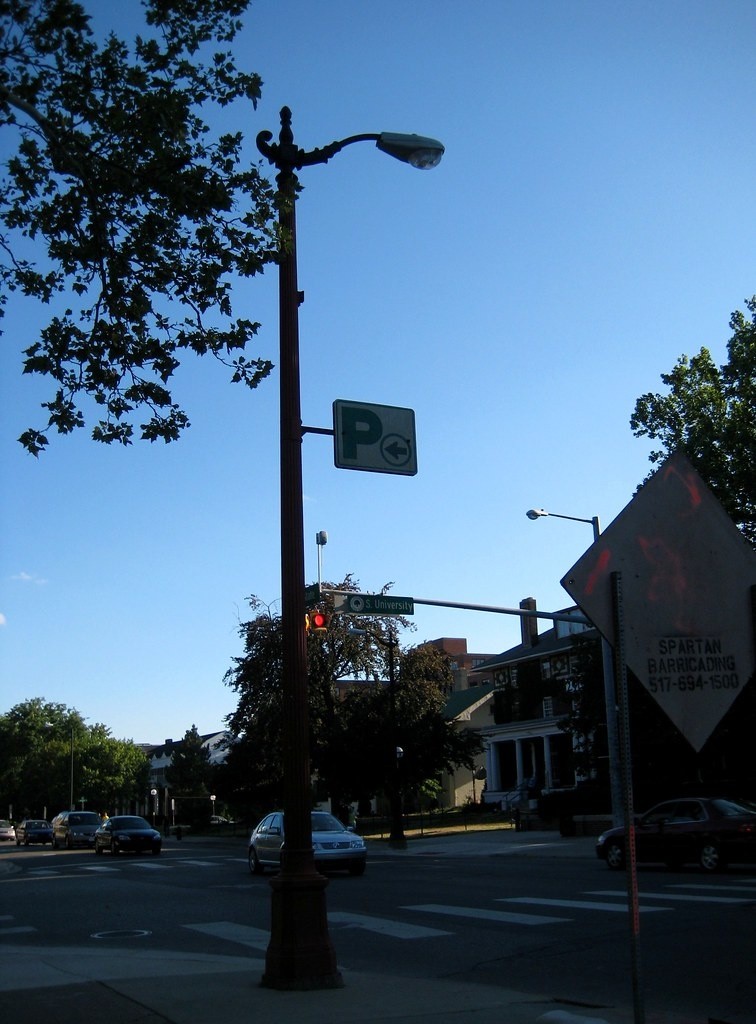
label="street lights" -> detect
[150,789,158,828]
[524,508,628,828]
[255,103,444,984]
[209,794,216,815]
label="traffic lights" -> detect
[309,612,328,631]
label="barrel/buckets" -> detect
[501,800,508,811]
[529,799,538,809]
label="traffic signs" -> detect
[332,398,418,477]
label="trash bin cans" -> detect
[341,806,355,830]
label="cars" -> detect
[594,796,756,874]
[209,815,235,826]
[248,811,367,876]
[94,815,162,856]
[51,812,69,825]
[15,819,53,848]
[0,819,16,841]
[51,811,105,850]
